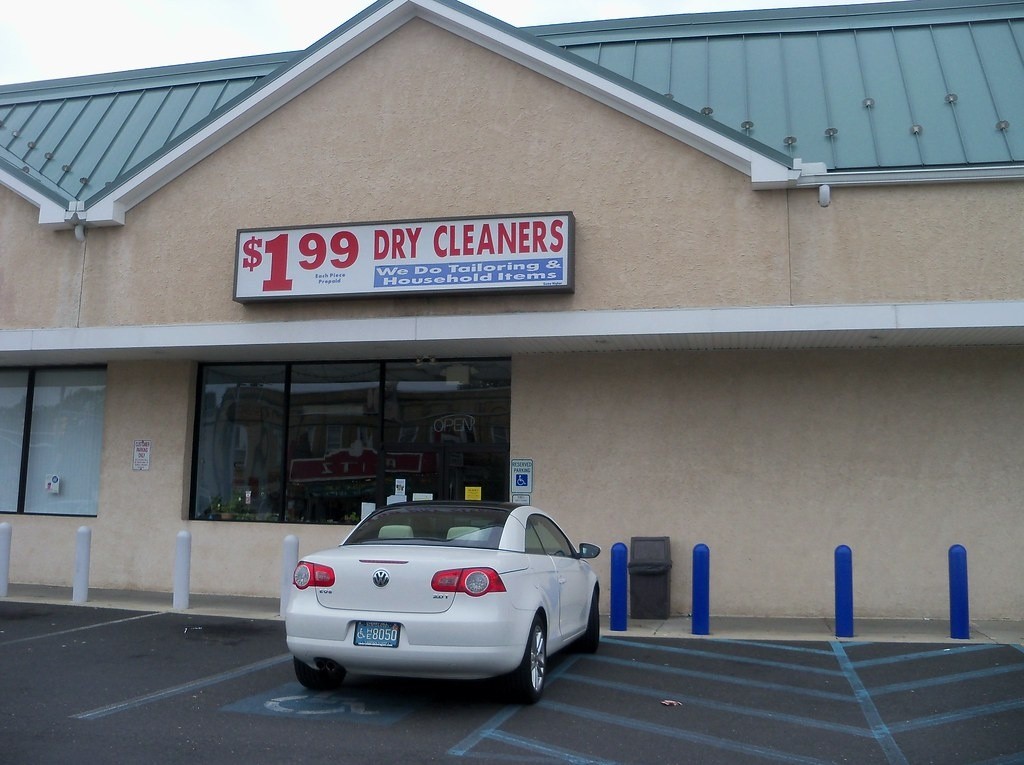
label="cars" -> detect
[282,499,606,700]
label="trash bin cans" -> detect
[626,535,673,618]
